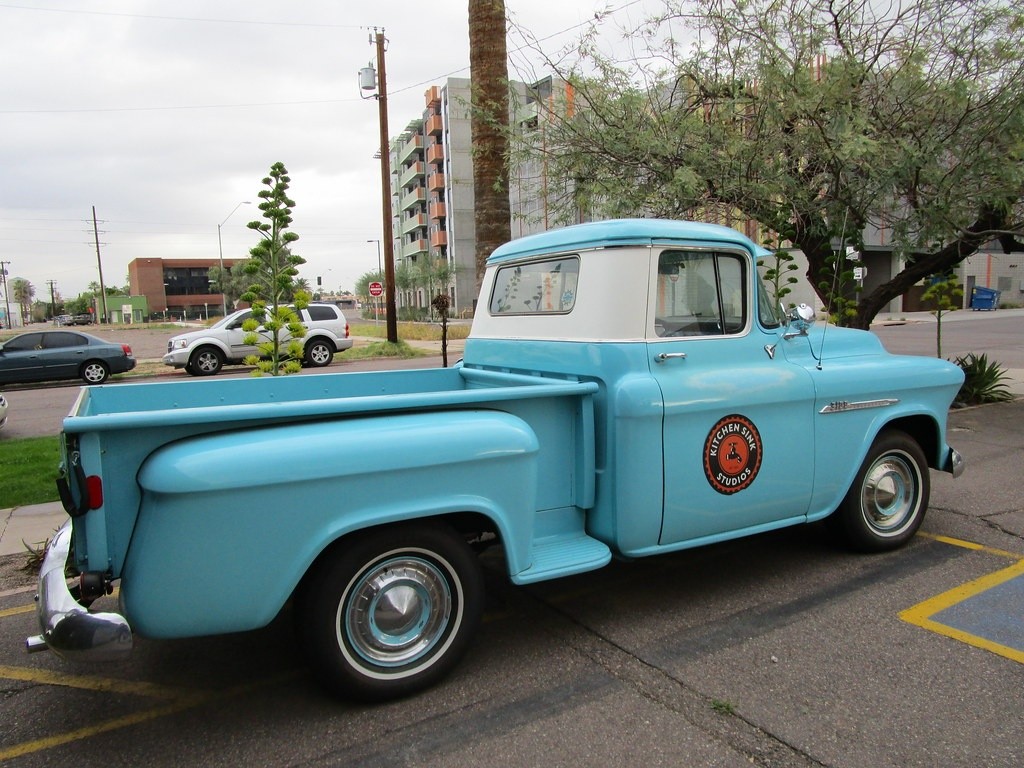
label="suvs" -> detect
[162,300,352,376]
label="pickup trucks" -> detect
[26,216,966,702]
[62,316,92,325]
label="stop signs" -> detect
[369,282,383,297]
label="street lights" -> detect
[217,201,252,318]
[366,239,383,315]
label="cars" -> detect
[0,328,138,385]
